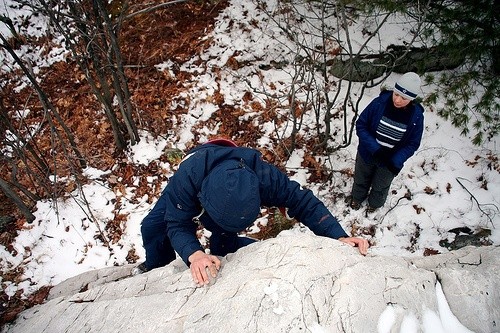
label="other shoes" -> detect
[349,199,361,210]
[365,206,377,218]
[133,262,151,275]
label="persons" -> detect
[349,72,424,214]
[138,138,370,286]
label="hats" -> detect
[394,72,422,102]
